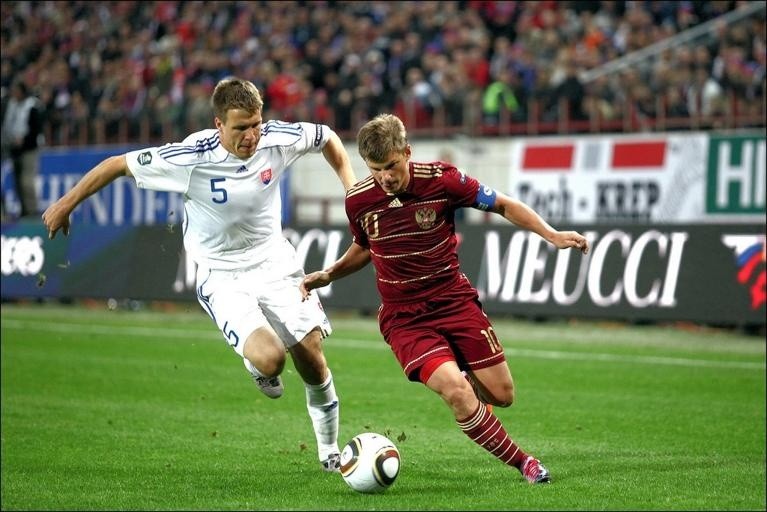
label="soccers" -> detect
[339,432,403,492]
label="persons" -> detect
[0,72,44,221]
[41,75,357,476]
[296,111,587,487]
[0,0,762,154]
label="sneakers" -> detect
[241,358,284,402]
[321,452,343,473]
[460,370,492,413]
[523,455,551,485]
